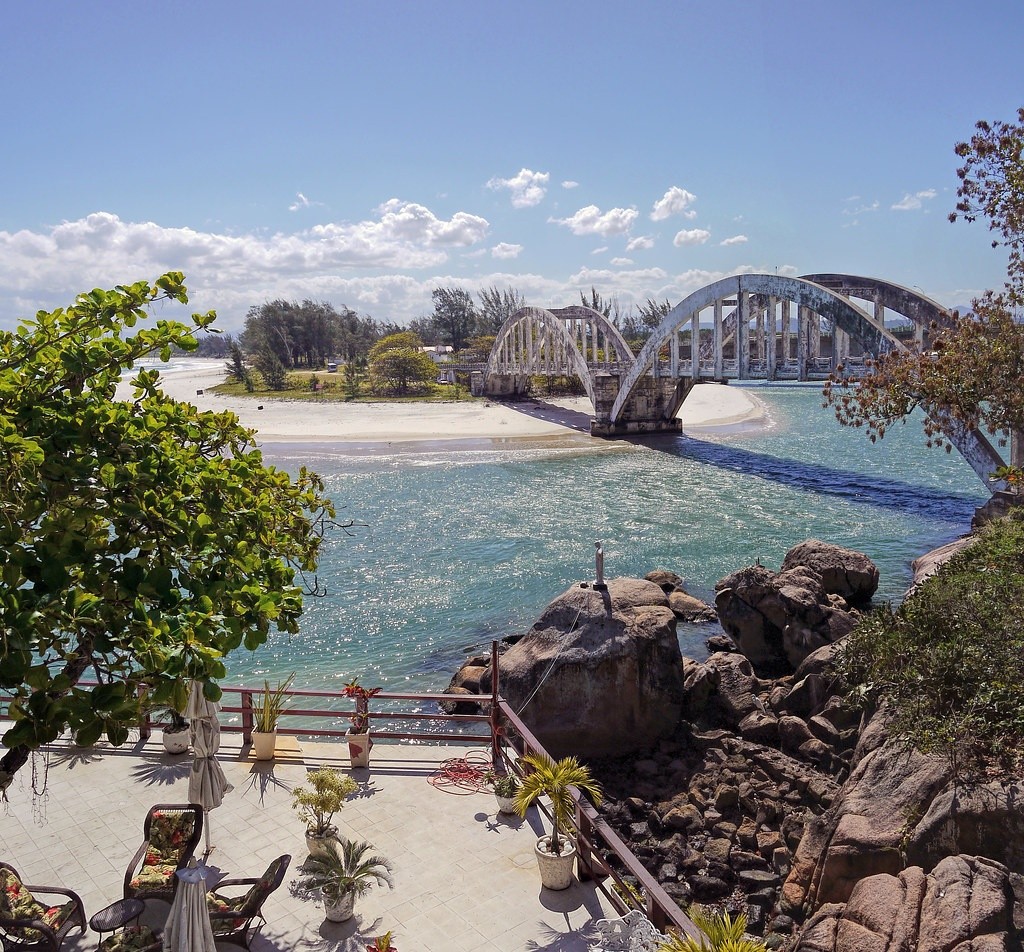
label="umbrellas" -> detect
[163,856,218,952]
[179,678,234,852]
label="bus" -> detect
[327,363,337,373]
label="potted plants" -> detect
[291,764,358,859]
[488,771,520,816]
[517,754,603,889]
[288,835,393,924]
[341,676,385,768]
[156,678,192,753]
[248,670,299,761]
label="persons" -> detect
[594,542,603,584]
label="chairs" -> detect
[0,863,87,952]
[94,925,163,952]
[123,804,204,904]
[206,855,292,952]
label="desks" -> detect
[89,898,145,947]
